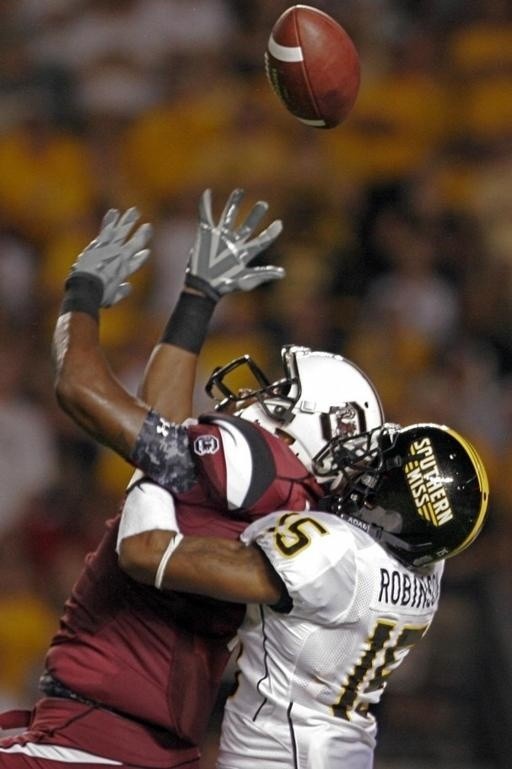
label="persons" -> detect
[0,184,386,769]
[1,171,512,618]
[111,420,489,769]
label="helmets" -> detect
[205,345,386,499]
[313,423,487,570]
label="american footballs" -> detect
[265,4,359,128]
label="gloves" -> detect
[184,187,287,303]
[64,207,154,308]
